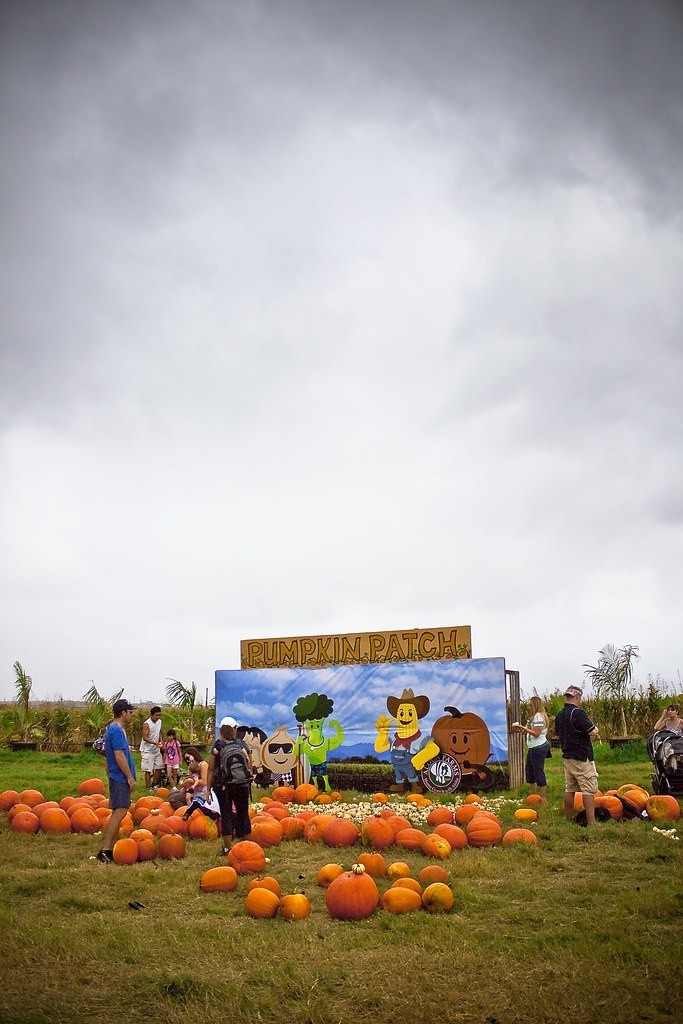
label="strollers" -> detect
[647,728,683,799]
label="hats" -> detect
[113,699,137,714]
[219,717,239,728]
[564,688,582,697]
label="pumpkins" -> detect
[0,777,219,866]
[199,783,544,919]
[562,784,680,822]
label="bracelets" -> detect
[127,777,133,780]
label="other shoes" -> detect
[152,785,159,790]
[219,845,231,856]
[146,786,153,791]
[171,786,178,791]
[97,850,114,865]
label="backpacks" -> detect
[217,739,254,802]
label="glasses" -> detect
[185,755,190,760]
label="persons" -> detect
[512,697,552,798]
[654,705,683,739]
[206,717,252,857]
[555,686,599,828]
[163,731,182,791]
[140,706,164,789]
[165,747,214,820]
[96,699,137,864]
